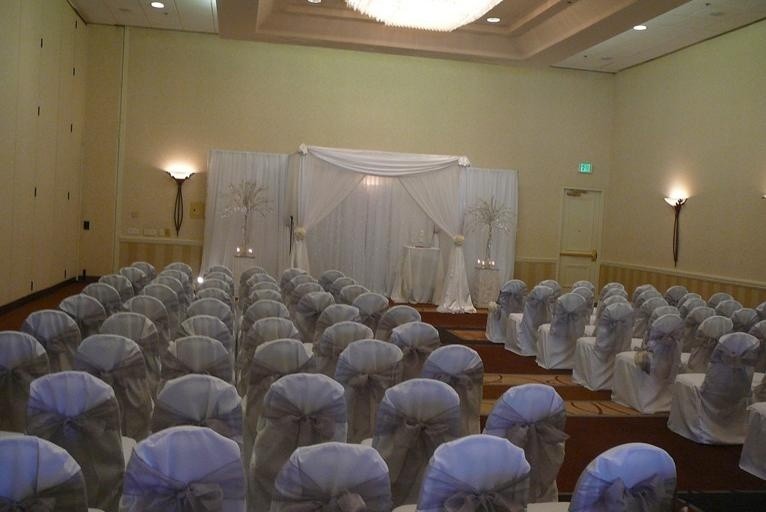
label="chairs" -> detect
[75,335,154,441]
[183,299,234,335]
[311,322,373,379]
[393,434,530,512]
[21,310,81,374]
[0,435,88,512]
[375,305,421,342]
[756,302,766,320]
[58,294,105,340]
[335,339,406,443]
[571,303,635,390]
[236,300,292,347]
[485,280,528,344]
[571,279,766,331]
[117,425,247,512]
[611,316,686,415]
[389,321,441,378]
[247,373,349,511]
[538,280,563,301]
[296,292,334,341]
[667,332,761,445]
[527,443,677,511]
[243,339,318,470]
[0,331,49,431]
[157,334,236,392]
[99,314,164,393]
[270,442,392,512]
[536,293,586,370]
[28,371,135,512]
[352,292,390,333]
[420,345,483,436]
[81,261,370,304]
[361,379,460,509]
[237,318,303,396]
[152,374,244,460]
[748,320,766,372]
[483,384,570,503]
[313,304,361,348]
[679,317,734,374]
[739,401,766,480]
[171,316,235,365]
[119,295,170,358]
[505,287,554,356]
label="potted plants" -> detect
[462,193,516,267]
[217,178,276,256]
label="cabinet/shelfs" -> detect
[473,267,500,308]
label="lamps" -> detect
[762,195,766,199]
[344,0,501,32]
[164,169,195,237]
[664,197,688,266]
